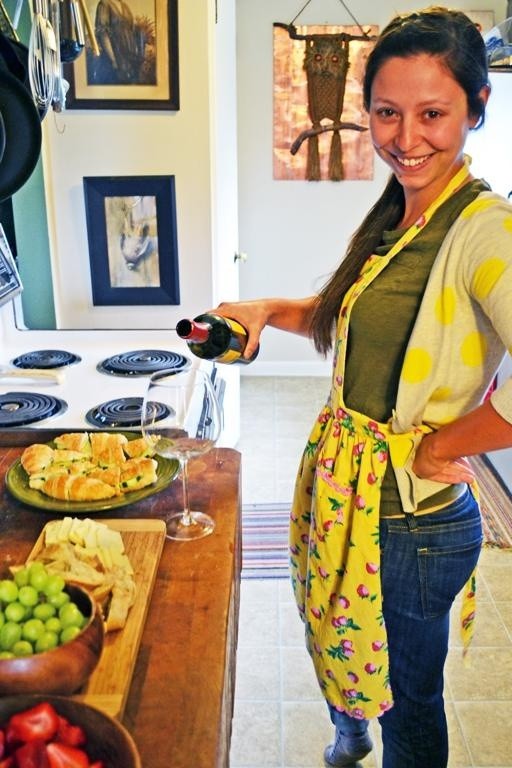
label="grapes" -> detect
[0,562,89,659]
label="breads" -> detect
[34,516,137,634]
[21,432,161,503]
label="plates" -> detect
[4,433,182,513]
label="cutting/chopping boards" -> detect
[20,517,168,723]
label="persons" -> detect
[206,6,512,768]
[95,0,134,85]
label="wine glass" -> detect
[141,366,223,542]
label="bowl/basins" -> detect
[0,694,143,768]
[2,583,104,699]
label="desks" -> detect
[0,425,245,767]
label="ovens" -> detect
[202,380,229,449]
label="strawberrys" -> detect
[0,701,105,768]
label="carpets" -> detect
[246,502,294,580]
[463,456,512,549]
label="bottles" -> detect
[177,315,260,366]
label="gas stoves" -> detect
[1,328,215,440]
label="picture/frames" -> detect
[86,175,182,311]
[66,1,183,113]
